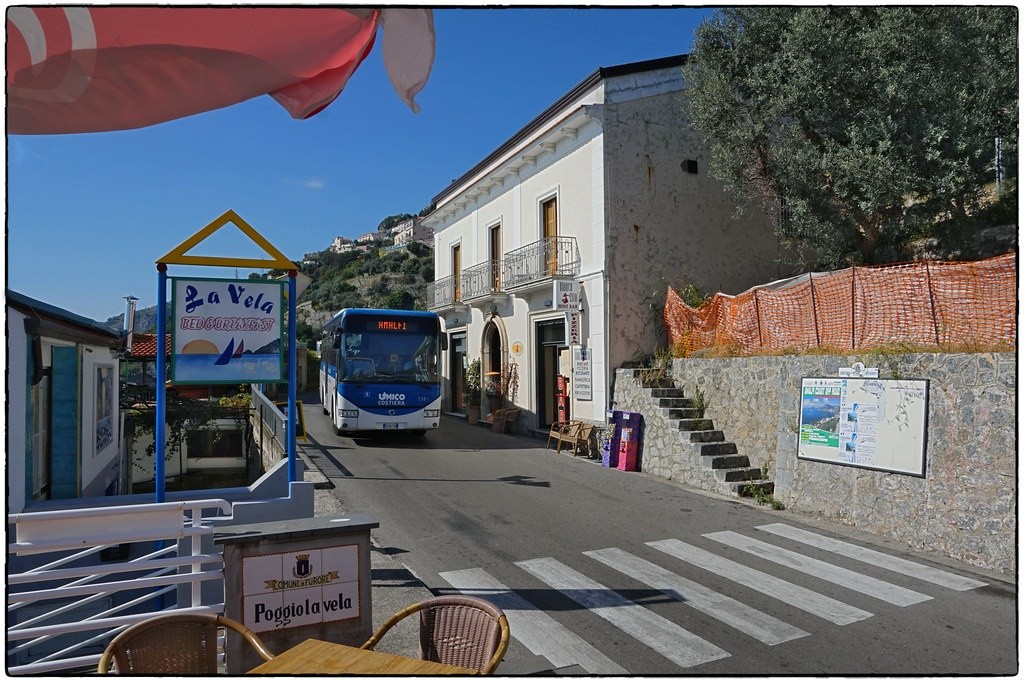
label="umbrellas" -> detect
[7,9,438,158]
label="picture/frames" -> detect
[795,376,931,479]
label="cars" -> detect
[166,380,223,402]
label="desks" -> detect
[245,638,481,675]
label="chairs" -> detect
[491,407,521,434]
[547,420,594,459]
[97,612,276,675]
[361,594,511,676]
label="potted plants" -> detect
[463,358,482,425]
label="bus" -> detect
[319,309,449,437]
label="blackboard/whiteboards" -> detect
[275,400,306,439]
